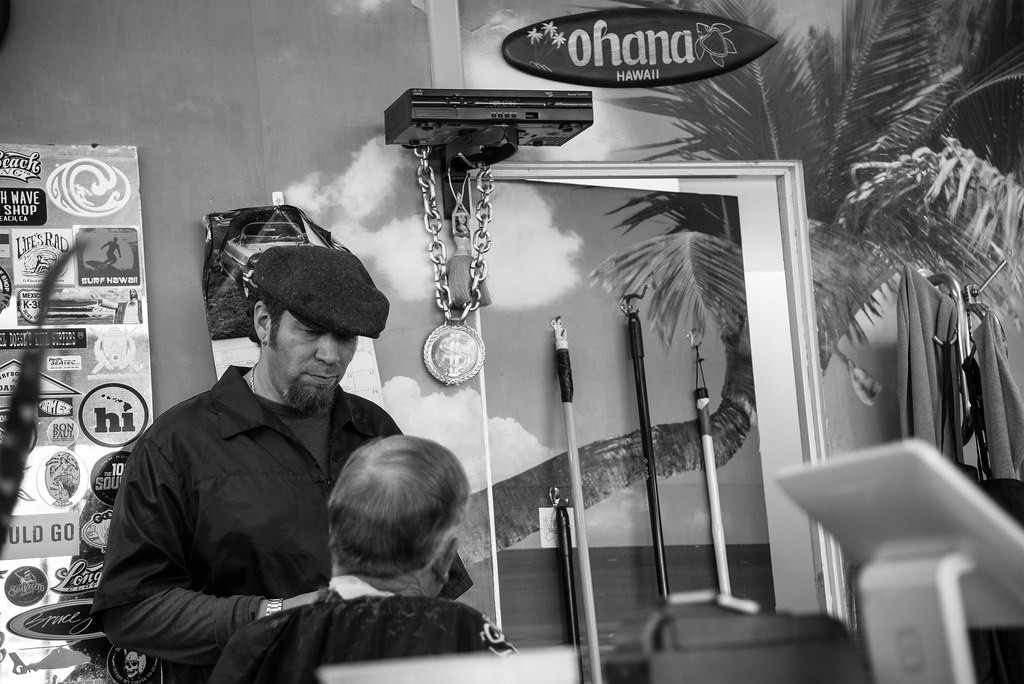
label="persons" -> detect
[211,435,519,684]
[91,246,473,684]
[90,289,143,324]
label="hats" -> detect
[250,245,390,339]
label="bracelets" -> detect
[266,598,283,617]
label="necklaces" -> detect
[250,363,257,393]
